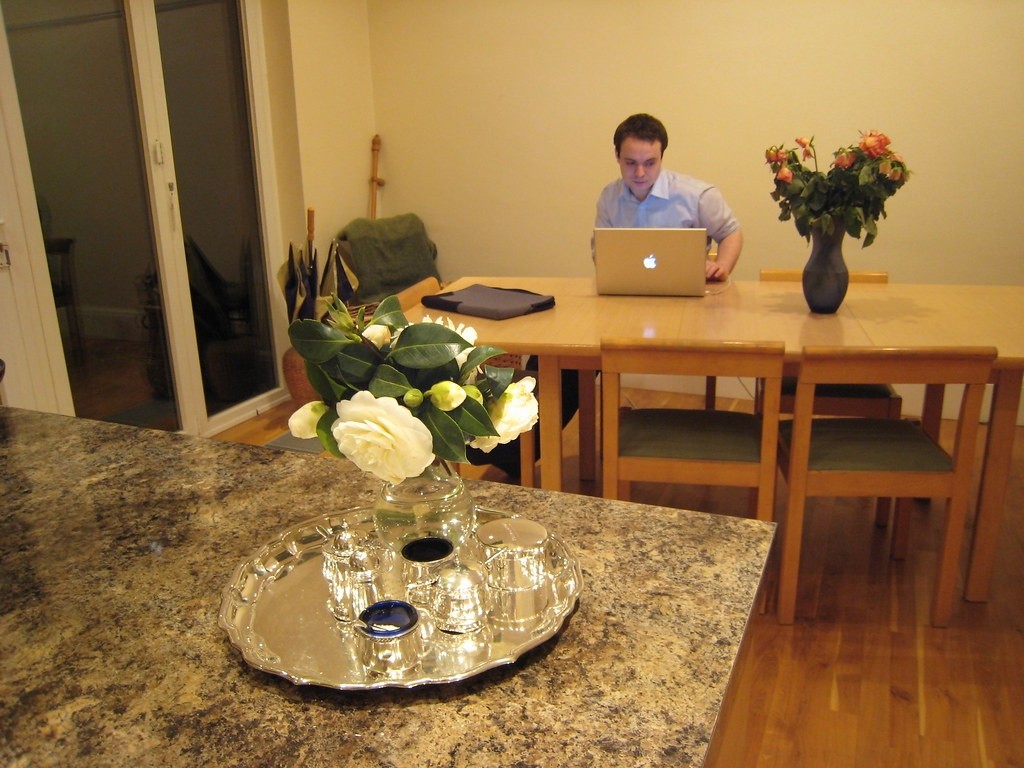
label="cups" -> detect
[321,520,393,624]
[474,518,555,631]
[400,536,459,610]
[428,556,486,637]
[348,548,387,627]
[351,601,434,677]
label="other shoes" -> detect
[465,445,521,482]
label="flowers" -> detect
[288,297,538,486]
[766,129,911,248]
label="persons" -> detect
[462,113,742,480]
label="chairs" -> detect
[756,268,902,421]
[602,336,999,629]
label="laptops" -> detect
[594,227,707,298]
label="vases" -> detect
[373,456,477,567]
[802,221,849,314]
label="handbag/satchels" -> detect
[420,284,555,320]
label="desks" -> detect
[401,276,1024,602]
[0,406,777,768]
